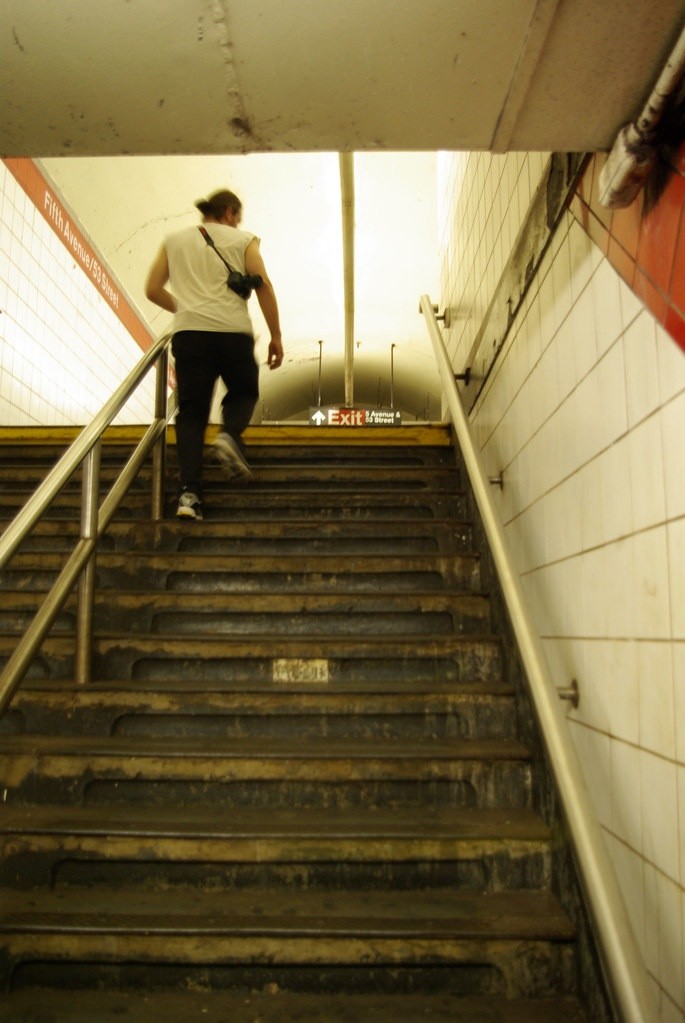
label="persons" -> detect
[147,190,283,521]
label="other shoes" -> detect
[177,494,205,521]
[206,432,256,485]
